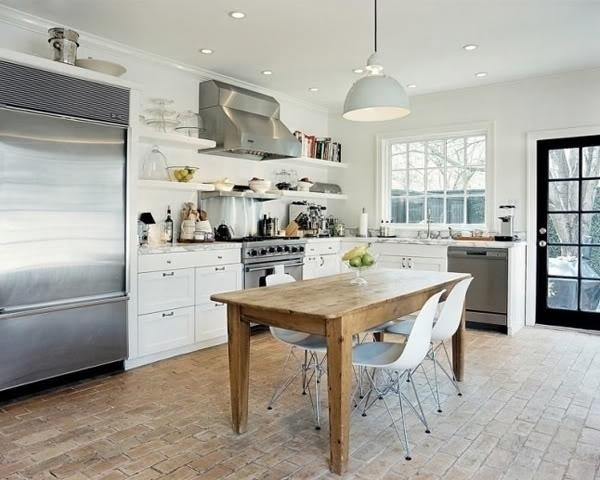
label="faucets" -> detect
[427,208,433,239]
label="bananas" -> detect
[342,246,369,259]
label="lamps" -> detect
[342,2,411,122]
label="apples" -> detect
[349,256,362,267]
[362,253,375,266]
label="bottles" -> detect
[260,215,279,236]
[326,218,346,236]
[380,220,394,236]
[295,132,341,161]
[164,206,173,243]
[358,208,368,236]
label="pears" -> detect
[174,169,179,176]
[176,173,183,179]
[179,169,182,172]
[185,174,192,180]
[181,170,188,176]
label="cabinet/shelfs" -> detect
[303,236,449,278]
[128,243,241,371]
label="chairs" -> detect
[387,274,474,414]
[257,273,326,430]
[351,286,447,459]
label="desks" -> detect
[210,268,472,475]
